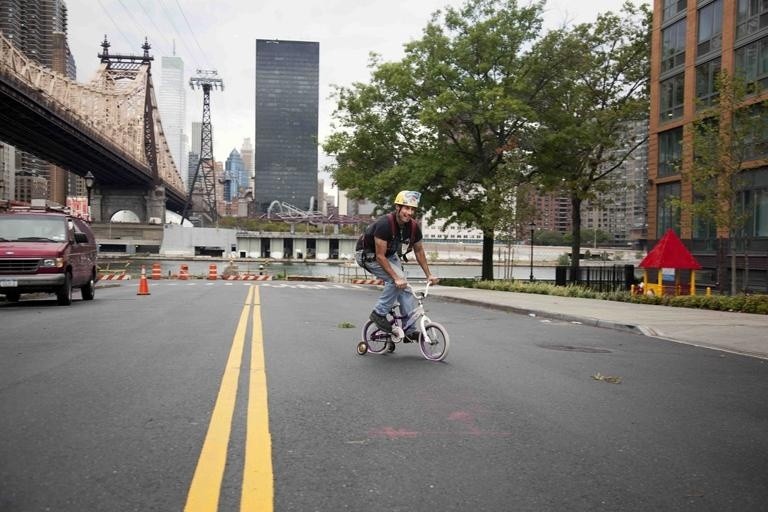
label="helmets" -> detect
[394,190,421,209]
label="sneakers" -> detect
[403,331,424,344]
[369,310,393,333]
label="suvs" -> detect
[0,205,98,304]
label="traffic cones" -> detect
[136,262,152,295]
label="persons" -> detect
[354,190,439,343]
